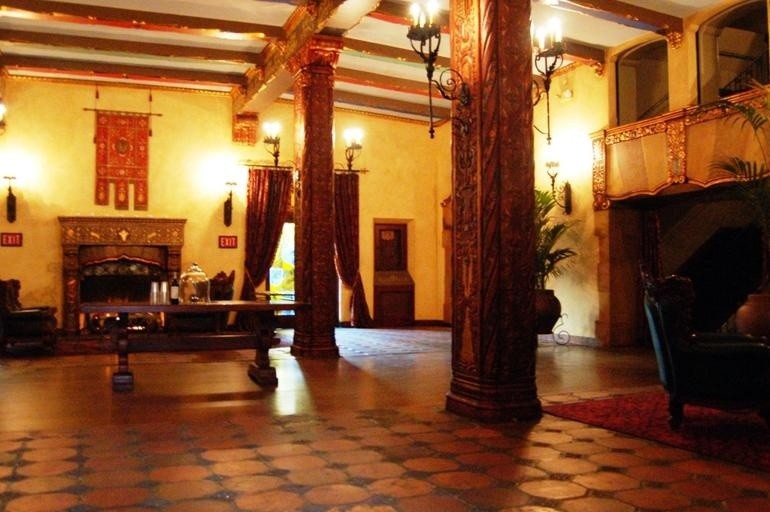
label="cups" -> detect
[149,281,159,305]
[159,281,169,305]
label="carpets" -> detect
[542,389,769,472]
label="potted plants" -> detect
[692,72,770,339]
[535,187,580,346]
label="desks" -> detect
[80,296,311,395]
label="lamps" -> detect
[404,2,469,140]
[531,16,569,148]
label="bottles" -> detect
[170,271,180,305]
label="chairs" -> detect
[1,279,59,356]
[639,271,769,429]
